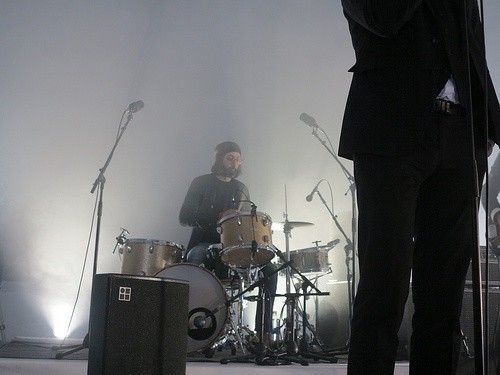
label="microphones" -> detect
[128,100,144,113]
[305,184,318,202]
[299,112,319,129]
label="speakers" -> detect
[87,273,191,375]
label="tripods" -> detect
[204,183,356,366]
[51,169,105,359]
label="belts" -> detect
[432,98,460,115]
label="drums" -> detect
[121,239,184,277]
[216,211,275,267]
[206,243,247,290]
[277,247,329,277]
[151,262,230,356]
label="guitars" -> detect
[485,216,500,259]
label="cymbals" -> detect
[271,222,314,230]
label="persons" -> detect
[339,1,500,375]
[481,151,500,226]
[179,141,279,344]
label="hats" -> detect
[214,142,241,154]
